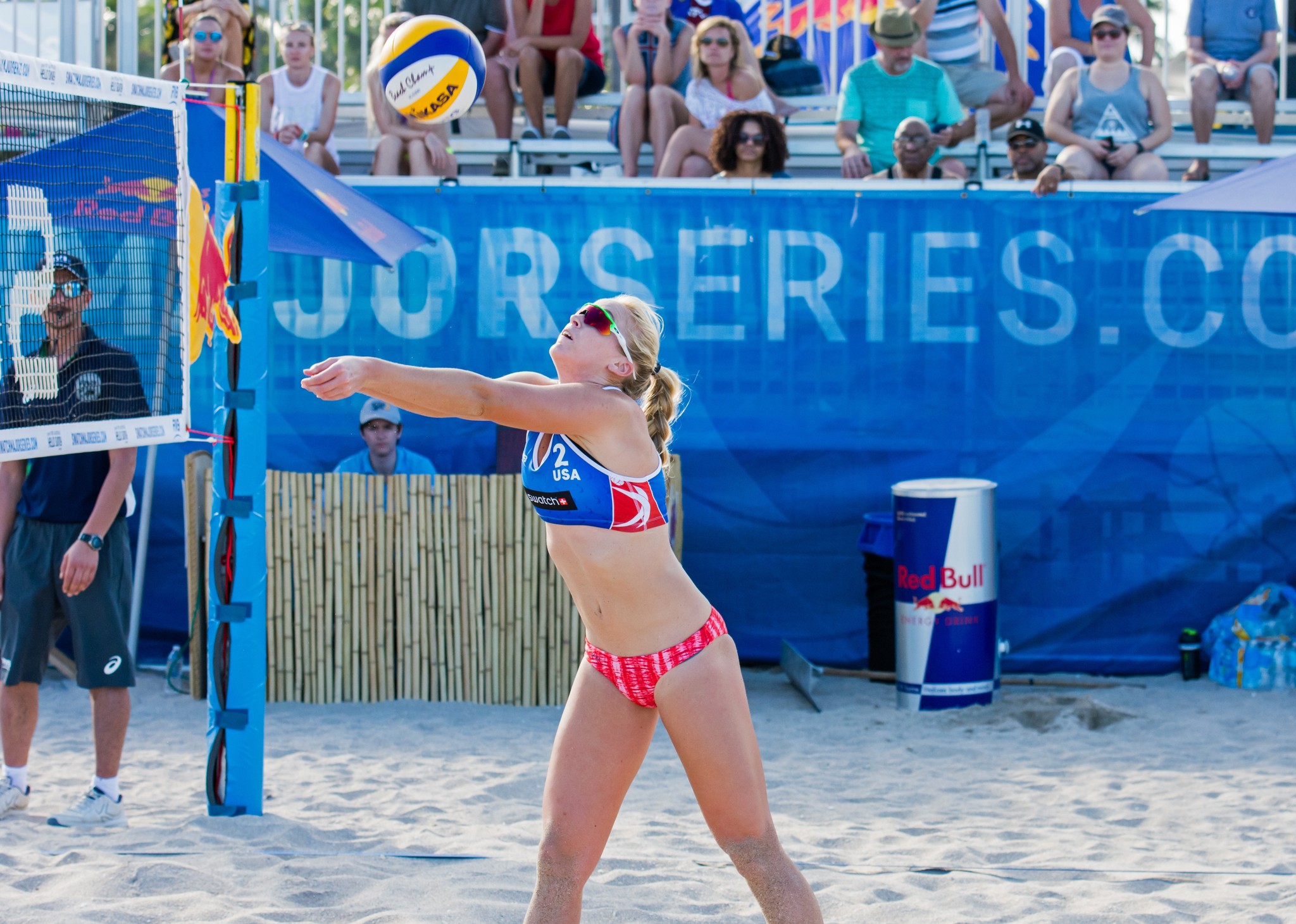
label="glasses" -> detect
[193,30,222,43]
[1095,29,1120,40]
[35,280,88,300]
[896,133,929,148]
[1011,139,1038,151]
[701,36,729,46]
[739,132,764,145]
[574,302,637,381]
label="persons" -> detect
[302,294,822,924]
[0,255,152,825]
[313,396,450,516]
[156,1,1282,197]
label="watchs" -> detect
[78,532,105,551]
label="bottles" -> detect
[164,645,182,694]
[1239,638,1296,690]
[1179,628,1204,680]
[1223,64,1238,81]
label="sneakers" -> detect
[47,785,129,828]
[0,776,31,820]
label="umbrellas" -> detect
[0,80,438,674]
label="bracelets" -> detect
[1052,163,1065,175]
[1133,140,1144,155]
[274,131,279,141]
[297,129,309,141]
[422,131,430,141]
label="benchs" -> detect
[0,82,1296,175]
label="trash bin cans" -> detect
[858,511,897,685]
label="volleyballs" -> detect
[377,13,487,126]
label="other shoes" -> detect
[521,126,544,158]
[492,156,509,176]
[552,125,571,158]
[534,163,554,175]
[1181,171,1210,182]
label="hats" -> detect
[1007,117,1044,142]
[35,253,88,280]
[359,398,401,425]
[1090,4,1128,29]
[867,8,922,47]
[761,34,802,69]
